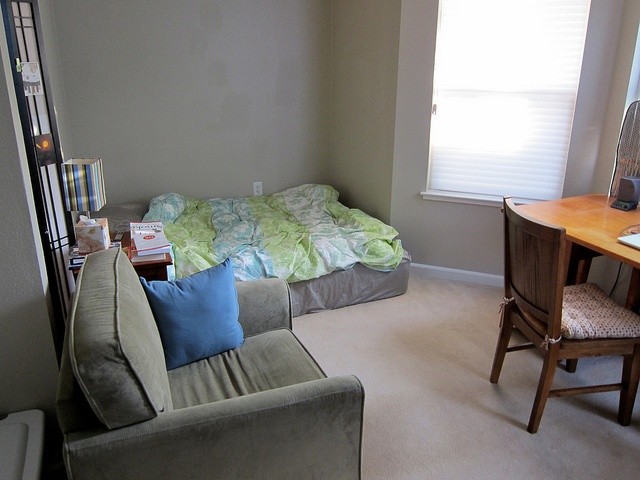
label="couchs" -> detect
[54,245,363,480]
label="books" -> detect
[128,220,172,262]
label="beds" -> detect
[83,183,410,317]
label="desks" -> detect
[512,193,640,271]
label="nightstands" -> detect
[72,232,173,281]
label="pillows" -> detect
[137,259,244,370]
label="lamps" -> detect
[62,158,108,219]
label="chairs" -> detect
[490,196,640,433]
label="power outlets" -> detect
[254,182,262,196]
